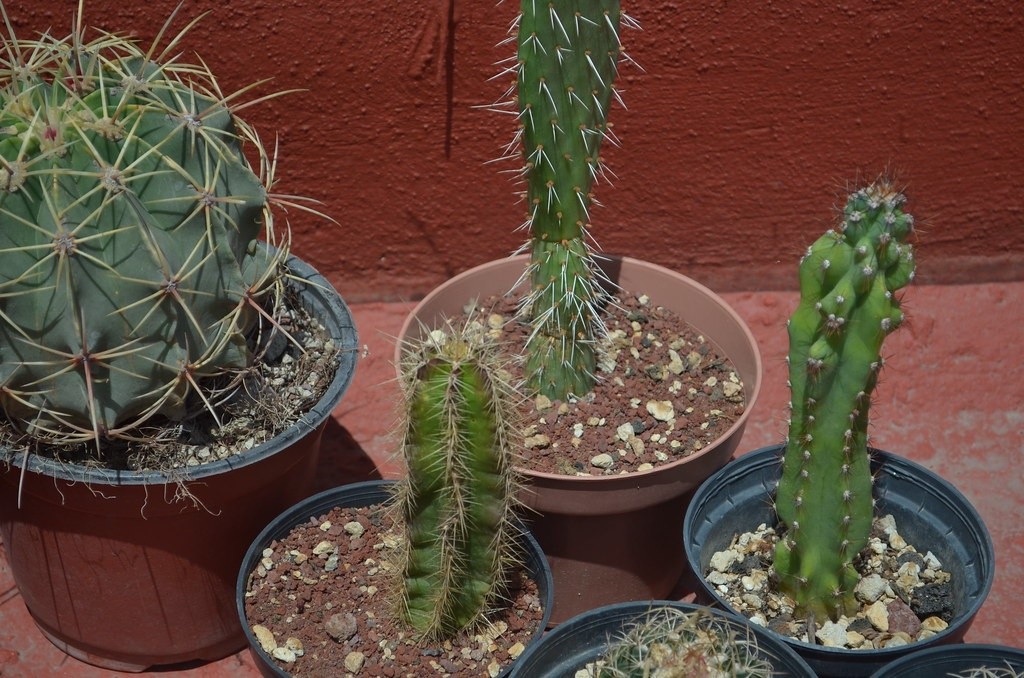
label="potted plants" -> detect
[0,0,361,676]
[506,599,820,678]
[868,642,1024,678]
[393,0,764,523]
[678,160,995,678]
[226,286,557,678]
